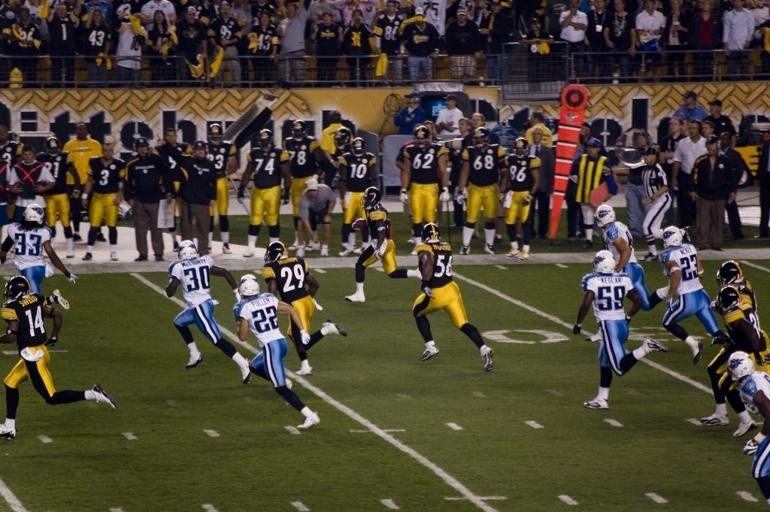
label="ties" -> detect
[535,145,539,156]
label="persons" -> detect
[659,226,728,366]
[674,91,708,121]
[311,0,374,9]
[753,139,770,241]
[402,7,440,84]
[141,0,176,26]
[202,123,237,254]
[658,116,687,224]
[114,9,149,82]
[301,177,336,257]
[448,117,472,228]
[80,9,113,83]
[0,204,79,310]
[587,205,669,342]
[397,121,442,168]
[237,129,291,258]
[42,0,83,88]
[498,137,541,261]
[461,113,500,148]
[604,0,635,83]
[700,287,770,437]
[243,12,279,82]
[311,10,343,87]
[753,20,770,80]
[584,0,612,84]
[716,133,742,239]
[700,122,714,138]
[394,92,424,135]
[374,0,405,88]
[188,1,219,22]
[165,240,250,384]
[153,128,192,252]
[570,138,619,248]
[671,120,708,227]
[176,6,209,79]
[320,110,353,189]
[690,135,737,252]
[525,112,553,148]
[516,0,566,43]
[572,250,668,410]
[251,0,274,26]
[122,137,176,262]
[666,0,691,82]
[435,94,463,135]
[455,127,509,255]
[723,0,755,79]
[636,0,666,78]
[479,0,515,85]
[400,124,451,254]
[277,0,312,86]
[0,159,12,226]
[704,100,737,149]
[412,223,496,372]
[208,3,242,86]
[36,137,81,258]
[728,351,770,510]
[339,138,378,255]
[565,125,590,241]
[603,148,671,262]
[560,0,589,82]
[234,280,321,430]
[345,186,423,304]
[0,125,23,167]
[1,7,43,85]
[0,275,117,440]
[81,137,127,262]
[146,9,178,80]
[156,135,216,257]
[6,145,55,223]
[282,119,327,251]
[260,241,347,376]
[709,260,761,332]
[63,122,107,242]
[443,9,482,82]
[625,132,652,238]
[344,9,375,87]
[691,0,721,82]
[526,127,553,238]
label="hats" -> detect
[136,138,149,148]
[705,136,719,144]
[404,92,418,99]
[306,179,318,191]
[193,141,206,149]
[682,91,697,97]
[442,94,457,100]
[457,9,467,16]
[415,7,425,16]
[708,99,722,107]
[640,145,657,154]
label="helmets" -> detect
[663,226,682,248]
[592,250,616,274]
[594,205,616,228]
[178,240,198,259]
[414,125,430,139]
[23,203,45,224]
[264,241,288,263]
[334,126,354,150]
[258,129,273,151]
[352,137,366,157]
[44,137,60,157]
[4,275,29,297]
[239,274,260,295]
[714,286,741,315]
[716,260,744,286]
[728,351,754,381]
[513,138,529,158]
[472,127,488,149]
[8,134,20,144]
[209,123,223,136]
[291,120,308,141]
[586,137,601,147]
[421,223,440,243]
[363,186,382,210]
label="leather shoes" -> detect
[134,256,147,261]
[155,255,163,261]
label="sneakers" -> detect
[505,249,521,258]
[341,242,350,249]
[51,289,70,310]
[484,243,497,255]
[185,351,203,369]
[638,252,659,262]
[582,240,593,248]
[82,252,92,260]
[73,235,82,242]
[92,383,119,410]
[512,254,529,262]
[700,414,730,427]
[345,294,366,303]
[417,267,423,280]
[584,398,609,409]
[312,241,321,251]
[692,341,704,365]
[323,319,348,338]
[321,244,329,257]
[66,249,75,258]
[296,246,306,257]
[305,238,314,252]
[408,238,416,244]
[480,344,495,373]
[460,244,472,255]
[241,358,252,385]
[680,225,693,244]
[288,241,299,251]
[223,244,232,254]
[338,249,353,257]
[421,344,439,361]
[111,251,119,261]
[586,331,604,343]
[285,378,292,406]
[0,423,17,439]
[732,419,758,438]
[97,232,107,242]
[352,245,370,254]
[641,336,669,353]
[296,413,320,429]
[295,367,312,376]
[243,247,255,257]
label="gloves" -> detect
[375,247,382,260]
[522,194,532,206]
[237,188,245,204]
[233,288,242,302]
[439,187,451,202]
[499,191,506,204]
[626,313,631,324]
[743,433,768,456]
[65,273,79,287]
[300,329,311,345]
[71,190,80,198]
[710,336,728,346]
[456,190,466,205]
[281,188,290,205]
[423,286,435,299]
[45,336,58,347]
[754,349,770,367]
[399,188,409,206]
[573,322,582,335]
[313,298,324,312]
[665,297,675,313]
[352,218,367,230]
